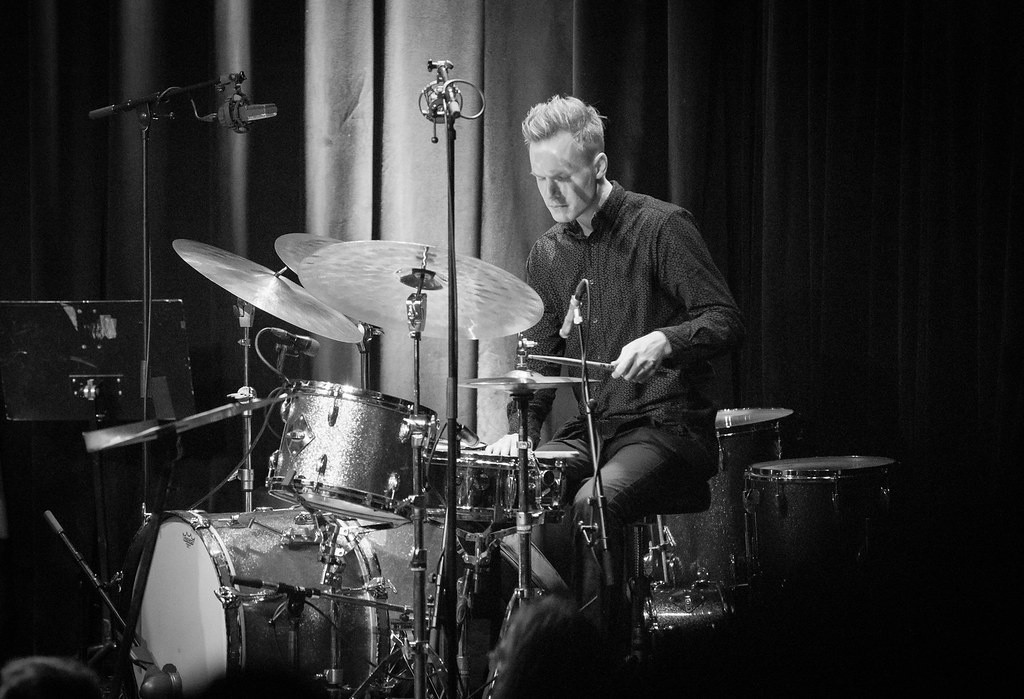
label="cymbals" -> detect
[171,230,364,347]
[457,367,605,393]
[296,234,547,342]
[273,231,345,273]
[93,394,285,456]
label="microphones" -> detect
[269,328,319,358]
[139,673,182,699]
[560,279,586,338]
[218,101,278,128]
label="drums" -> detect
[263,373,441,526]
[349,517,573,699]
[419,444,586,529]
[742,450,909,589]
[113,487,392,699]
[647,402,802,590]
[631,577,738,683]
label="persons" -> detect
[483,95,749,699]
[0,578,614,699]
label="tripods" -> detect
[350,271,446,699]
